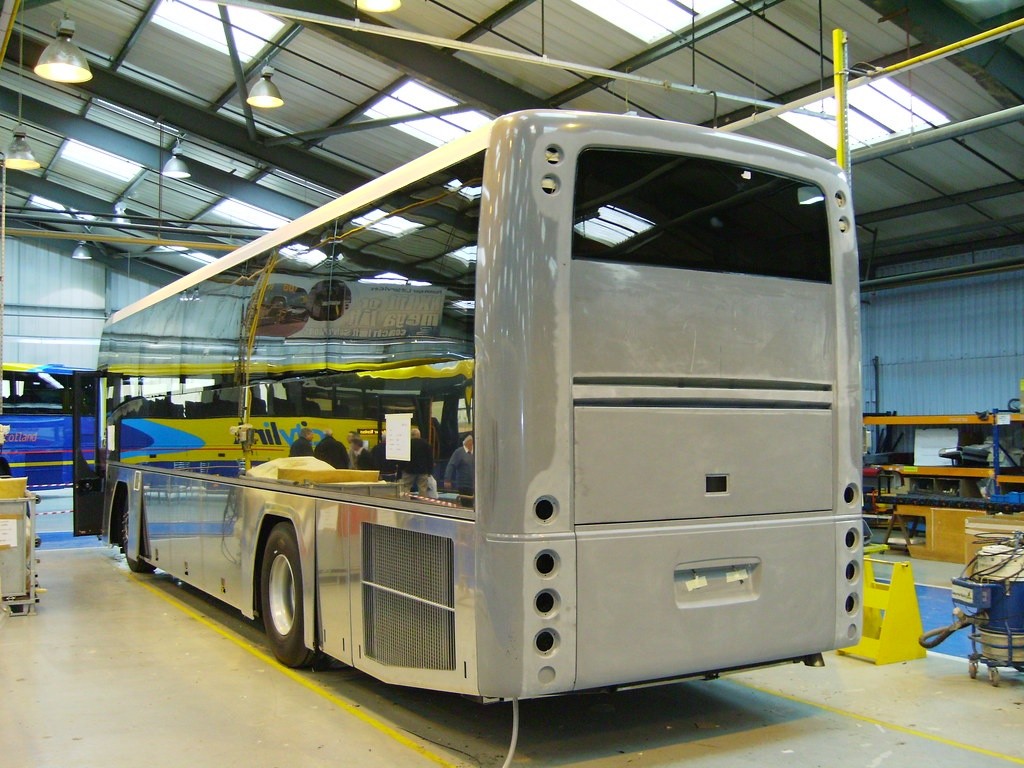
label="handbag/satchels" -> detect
[426,475,439,500]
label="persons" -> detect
[444,435,475,508]
[289,428,433,496]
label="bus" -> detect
[0,355,476,550]
[70,110,864,709]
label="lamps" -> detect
[245,64,285,108]
[4,125,41,171]
[162,137,192,180]
[112,202,133,225]
[33,11,93,84]
[180,287,201,301]
[70,240,93,260]
